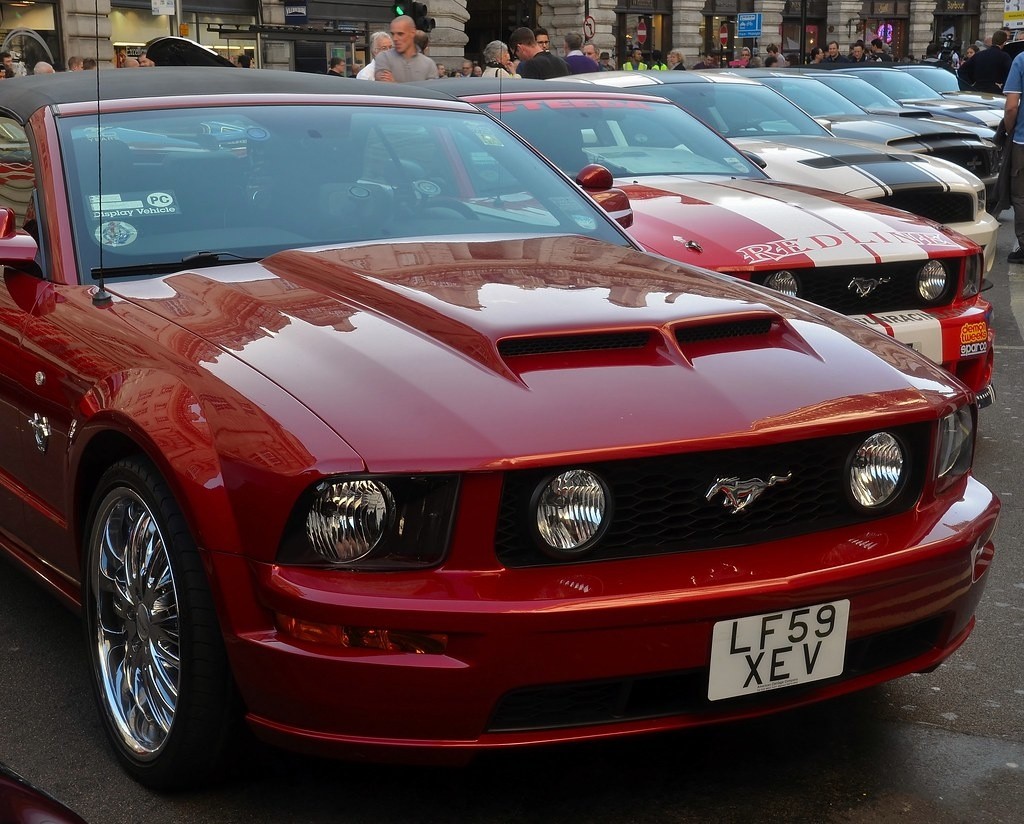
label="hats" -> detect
[600,52,609,59]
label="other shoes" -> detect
[1007,248,1024,262]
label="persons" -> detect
[0,16,1024,97]
[1004,52,1024,264]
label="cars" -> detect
[0,64,1003,795]
[540,58,1022,297]
[289,73,996,415]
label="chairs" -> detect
[265,149,393,243]
[73,139,185,245]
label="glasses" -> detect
[462,66,470,70]
[740,53,749,57]
[513,42,522,58]
[536,41,550,46]
[374,44,393,51]
